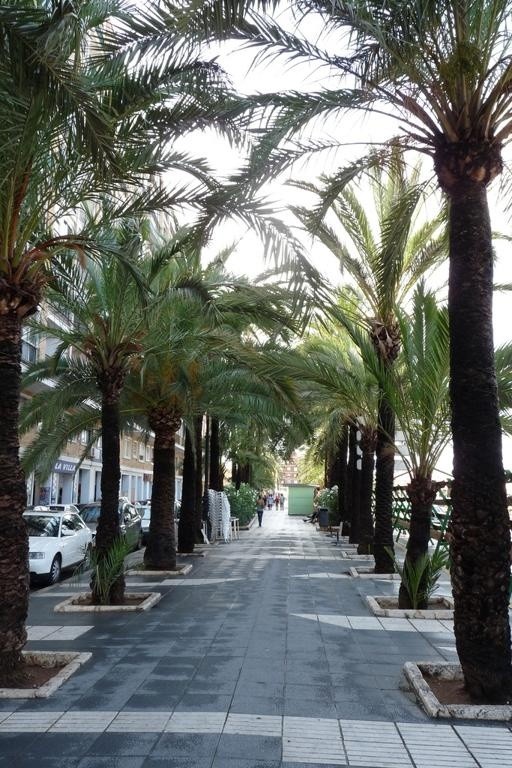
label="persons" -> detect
[256,494,264,527]
[262,492,285,510]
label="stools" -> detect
[229,517,240,541]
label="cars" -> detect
[22,498,182,588]
